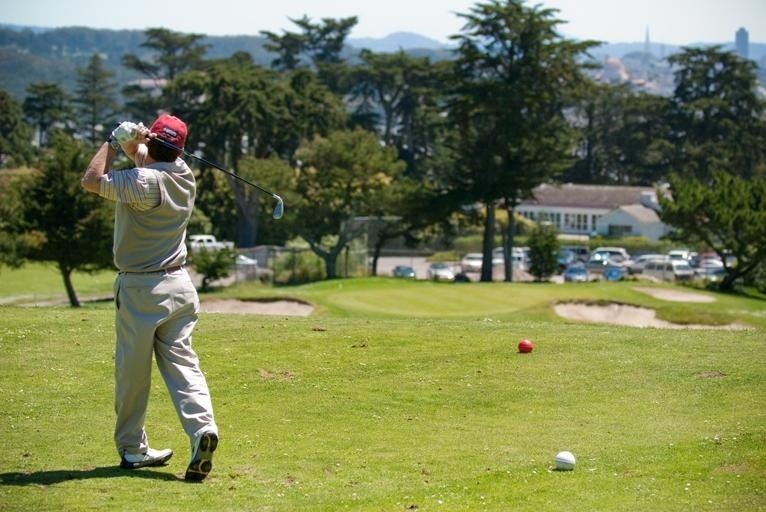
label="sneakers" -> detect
[121,447,172,468]
[184,430,218,483]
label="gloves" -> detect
[111,119,138,145]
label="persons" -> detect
[82,113,219,481]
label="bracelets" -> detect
[104,133,120,151]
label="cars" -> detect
[493,245,722,284]
[426,261,455,281]
[392,267,416,280]
[460,252,484,275]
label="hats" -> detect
[150,113,187,152]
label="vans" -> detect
[188,233,217,245]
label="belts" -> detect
[118,264,187,275]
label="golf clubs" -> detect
[117,122,283,220]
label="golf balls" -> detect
[518,339,532,351]
[555,451,574,471]
